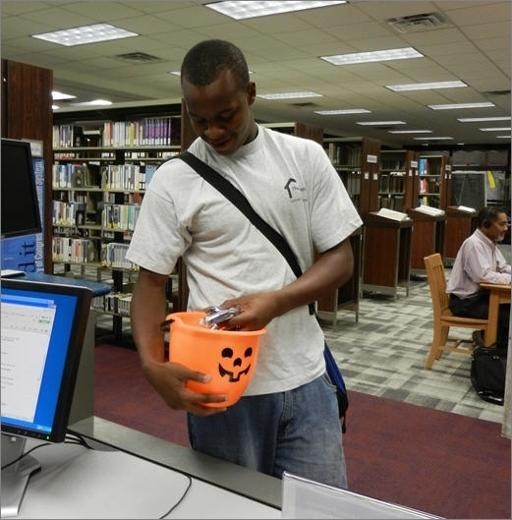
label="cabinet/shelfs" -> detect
[48,98,197,349]
[261,121,481,329]
[0,58,54,275]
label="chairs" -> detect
[421,253,488,369]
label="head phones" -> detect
[483,206,492,228]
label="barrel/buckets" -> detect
[166,311,266,408]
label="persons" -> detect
[445,206,512,348]
[124,40,364,488]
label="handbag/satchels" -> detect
[470,340,506,406]
[324,344,349,419]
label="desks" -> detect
[478,282,511,354]
[2,417,283,519]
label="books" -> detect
[324,143,430,212]
[52,116,179,317]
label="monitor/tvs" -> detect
[0,278,93,517]
[1,139,43,239]
